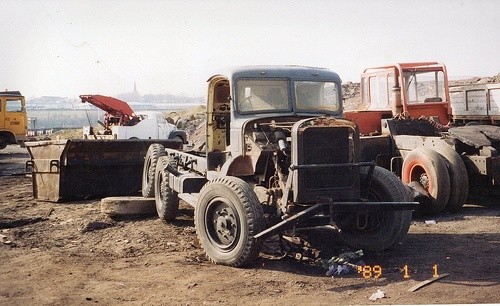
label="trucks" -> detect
[140,64,420,268]
[343,61,500,217]
[0,91,28,150]
[448,84,500,126]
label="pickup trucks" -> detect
[82,111,189,150]
